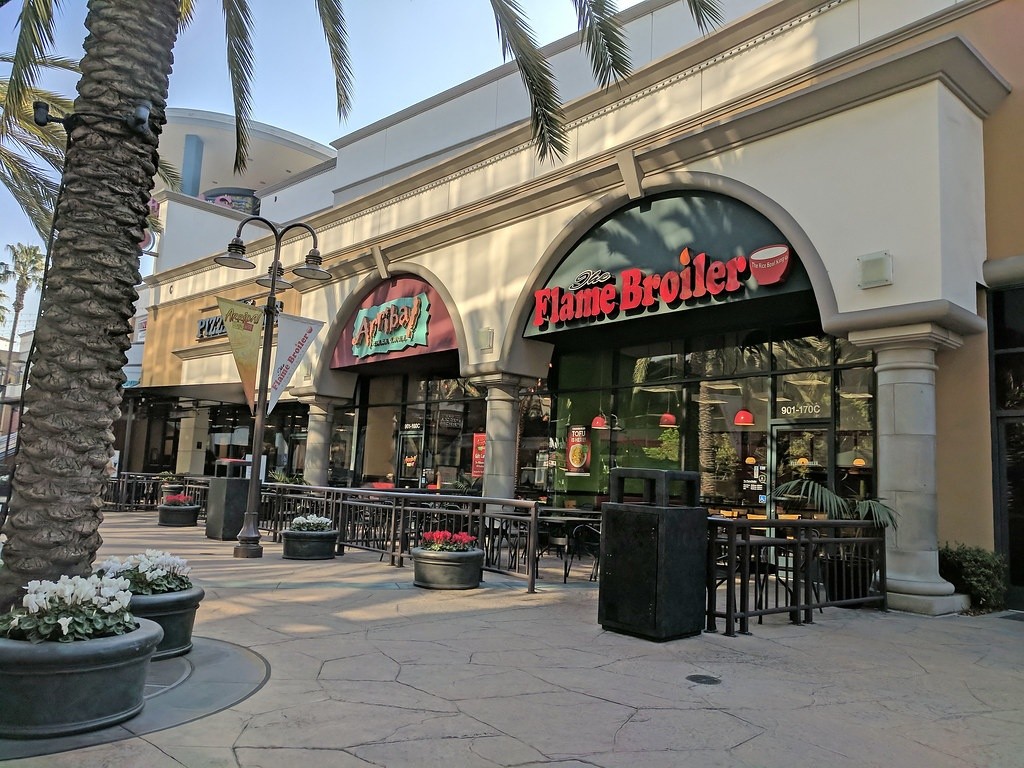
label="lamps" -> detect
[592,350,611,430]
[733,332,754,425]
[659,341,677,427]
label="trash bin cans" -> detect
[206,458,263,541]
[597,466,708,643]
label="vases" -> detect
[157,504,201,527]
[411,547,485,590]
[0,615,164,741]
[131,585,205,662]
[279,529,340,560]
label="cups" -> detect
[405,486,410,489]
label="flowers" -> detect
[0,574,136,645]
[91,548,192,595]
[419,530,478,552]
[166,495,193,506]
[289,514,332,532]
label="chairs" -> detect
[346,499,823,625]
[267,490,300,535]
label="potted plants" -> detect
[765,471,902,610]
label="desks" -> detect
[487,506,599,579]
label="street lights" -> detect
[213,215,333,560]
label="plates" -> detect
[568,444,587,467]
